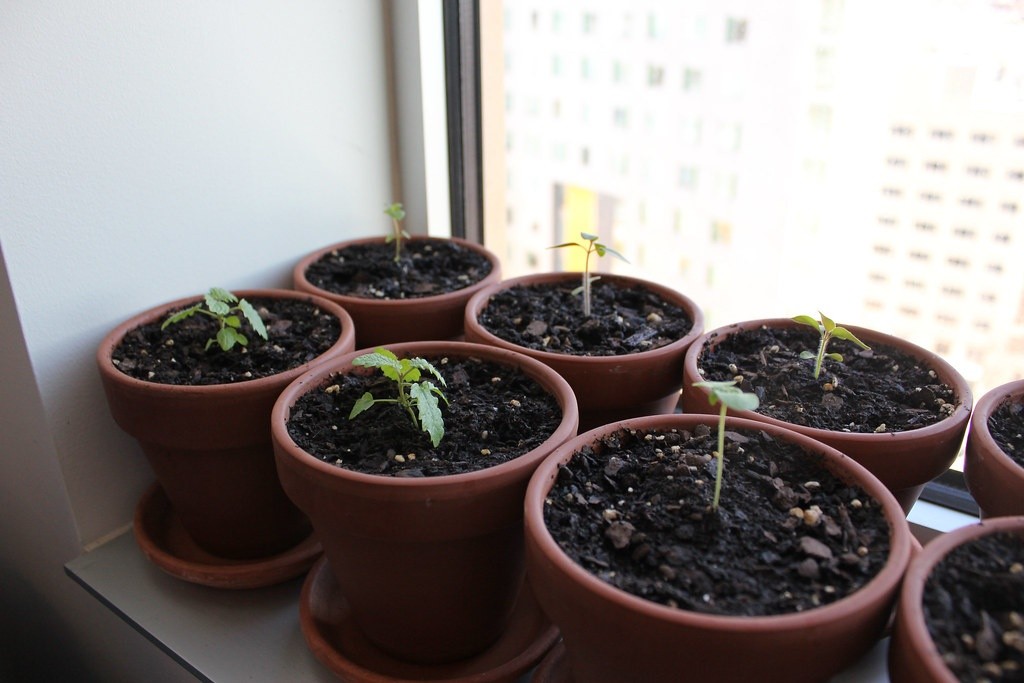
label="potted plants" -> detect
[97,201,971,683]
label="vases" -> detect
[963,378,1024,520]
[888,514,1024,683]
